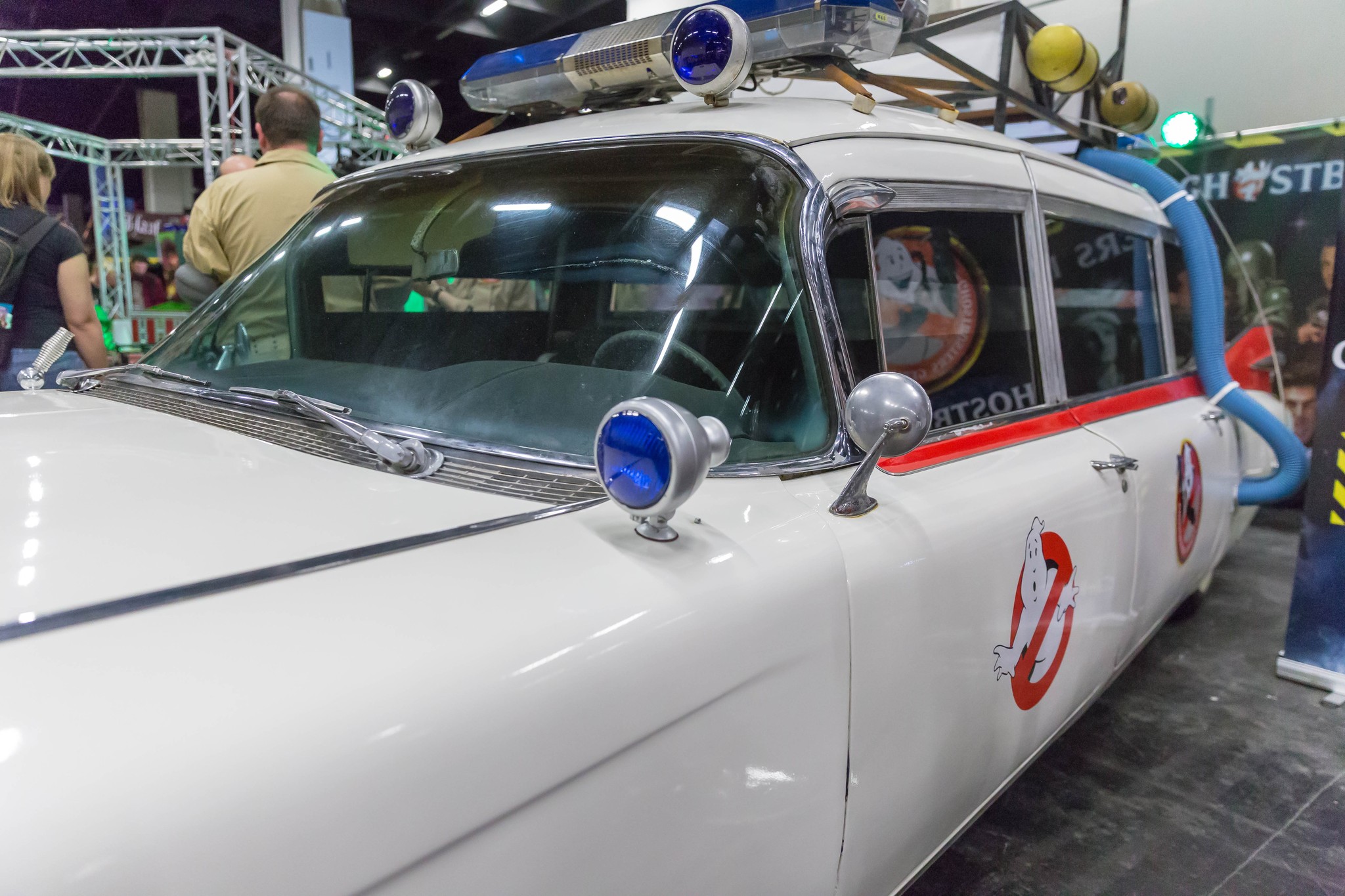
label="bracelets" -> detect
[432,286,444,303]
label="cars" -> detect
[0,0,1312,896]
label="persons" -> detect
[90,262,113,306]
[0,130,110,393]
[130,255,168,309]
[160,242,183,301]
[1074,306,1130,391]
[409,278,535,311]
[1271,242,1336,437]
[183,85,341,283]
[215,155,258,180]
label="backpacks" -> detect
[0,215,59,368]
